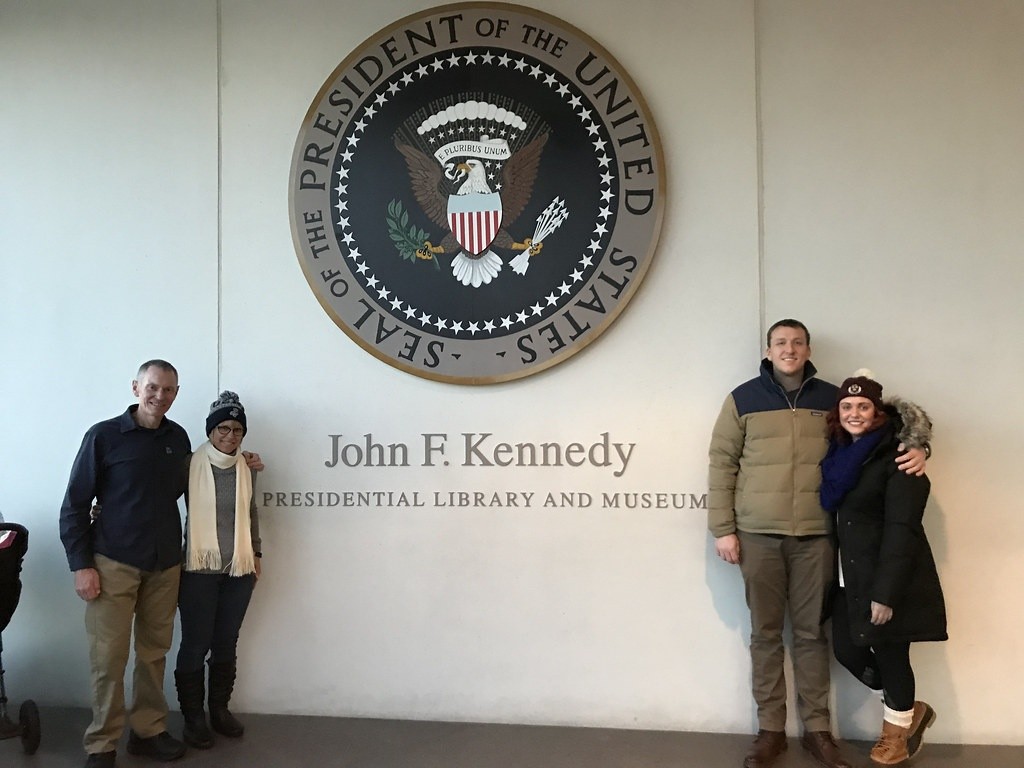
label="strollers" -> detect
[1,523,42,756]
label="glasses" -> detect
[218,426,245,436]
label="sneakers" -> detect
[85,750,116,768]
[127,728,186,761]
[870,701,936,765]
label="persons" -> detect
[816,369,948,765]
[91,390,262,750]
[708,319,931,768]
[60,357,266,768]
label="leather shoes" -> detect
[743,729,787,768]
[803,730,851,768]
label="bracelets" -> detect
[923,445,930,458]
[255,552,262,558]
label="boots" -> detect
[206,656,244,737]
[174,665,215,749]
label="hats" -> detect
[206,391,246,438]
[839,368,883,409]
